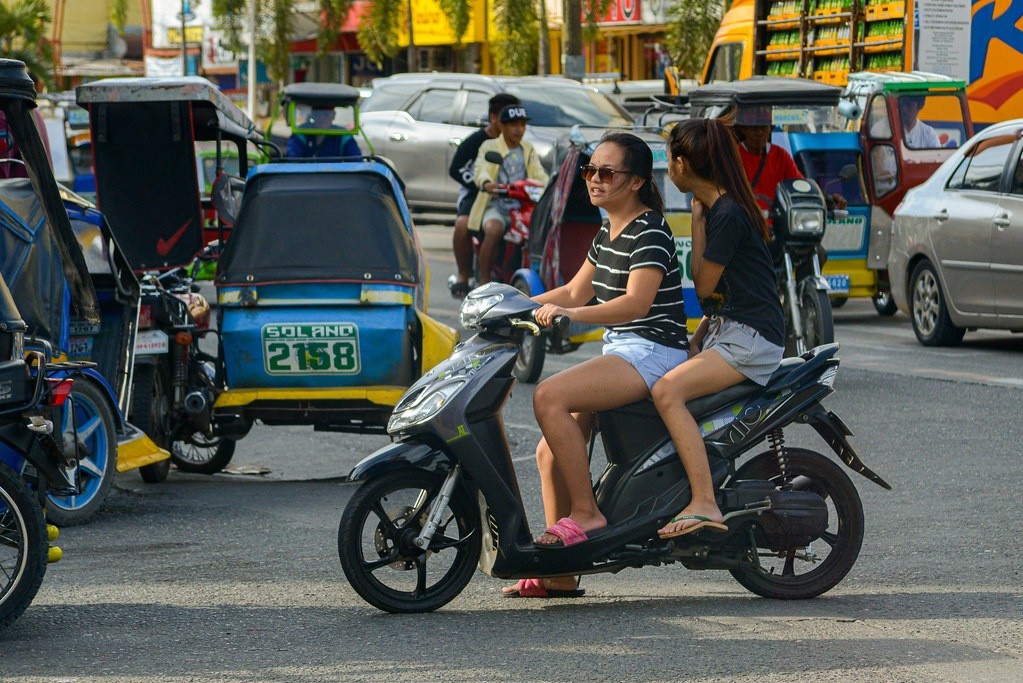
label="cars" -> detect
[884,119,1023,349]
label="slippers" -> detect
[501,576,585,598]
[533,517,613,549]
[658,515,729,539]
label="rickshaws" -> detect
[0,53,462,637]
[640,63,978,319]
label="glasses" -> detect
[579,164,635,183]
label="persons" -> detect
[449,94,523,291]
[869,89,939,197]
[467,105,551,285]
[287,106,362,162]
[736,104,847,232]
[649,118,785,538]
[501,132,690,598]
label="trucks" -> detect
[662,0,1023,148]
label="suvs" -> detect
[342,70,637,227]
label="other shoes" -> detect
[451,284,469,299]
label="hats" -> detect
[500,105,530,123]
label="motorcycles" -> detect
[334,281,894,615]
